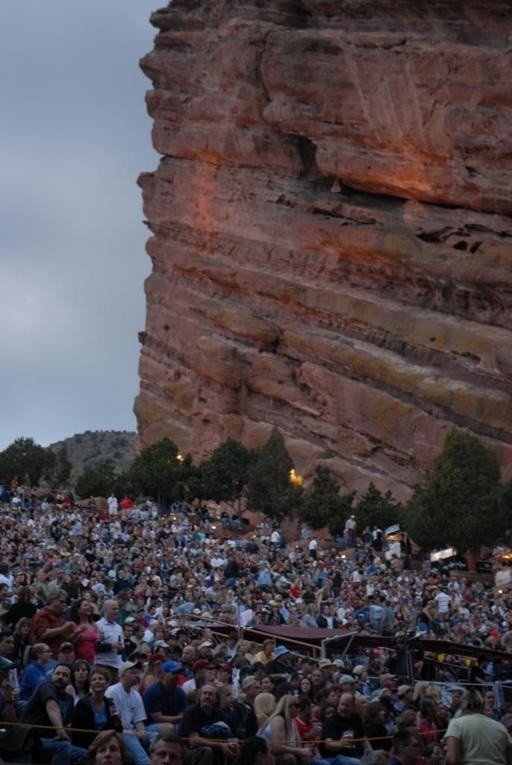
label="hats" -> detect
[1,608,438,713]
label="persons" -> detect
[0,475,512,765]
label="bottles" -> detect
[117,635,123,654]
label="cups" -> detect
[343,732,353,746]
[229,738,238,753]
